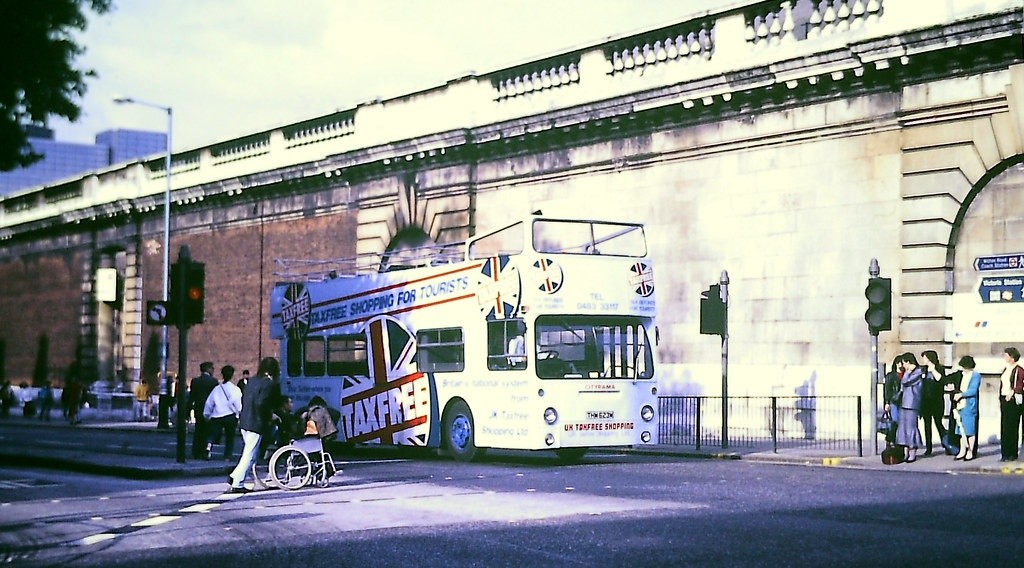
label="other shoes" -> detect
[1000,454,1009,462]
[902,454,909,462]
[223,455,233,462]
[204,449,211,460]
[226,475,234,485]
[227,487,251,493]
[924,450,932,456]
[1008,454,1018,461]
[907,455,916,463]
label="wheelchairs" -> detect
[252,418,343,492]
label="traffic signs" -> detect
[978,275,1024,304]
[973,253,1024,272]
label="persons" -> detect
[19,382,33,417]
[227,357,280,493]
[203,365,243,461]
[61,377,87,424]
[0,379,14,417]
[884,355,905,421]
[998,347,1024,462]
[896,352,924,463]
[186,362,219,458]
[279,396,333,471]
[136,380,152,422]
[39,381,54,421]
[951,355,981,461]
[920,350,947,455]
[237,370,250,388]
[509,335,540,362]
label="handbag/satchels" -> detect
[229,401,239,418]
[1013,366,1023,405]
[882,441,905,465]
[876,410,897,435]
[892,391,903,406]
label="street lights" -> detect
[113,95,174,432]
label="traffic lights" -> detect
[699,284,727,336]
[167,259,207,326]
[863,277,892,336]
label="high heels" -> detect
[964,451,974,461]
[954,452,967,461]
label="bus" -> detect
[269,216,661,465]
